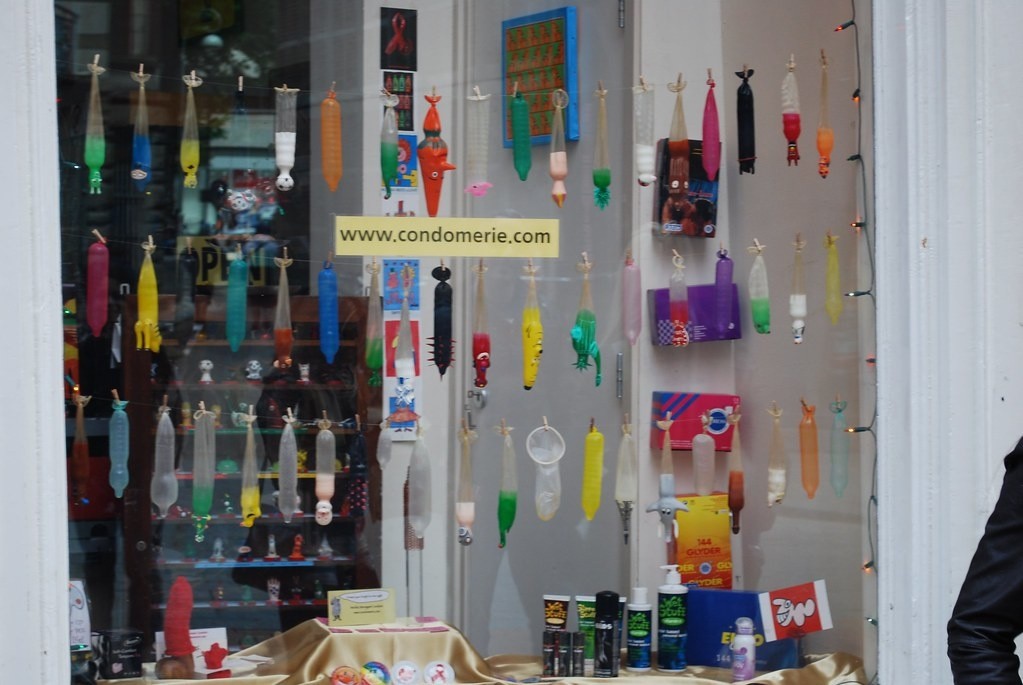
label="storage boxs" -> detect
[647,282,742,346]
[667,490,733,590]
[650,391,740,451]
[652,137,722,237]
[687,586,804,670]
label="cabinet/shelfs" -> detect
[123,293,385,654]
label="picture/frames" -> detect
[501,7,580,149]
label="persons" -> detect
[255,284,393,685]
[65,272,185,632]
